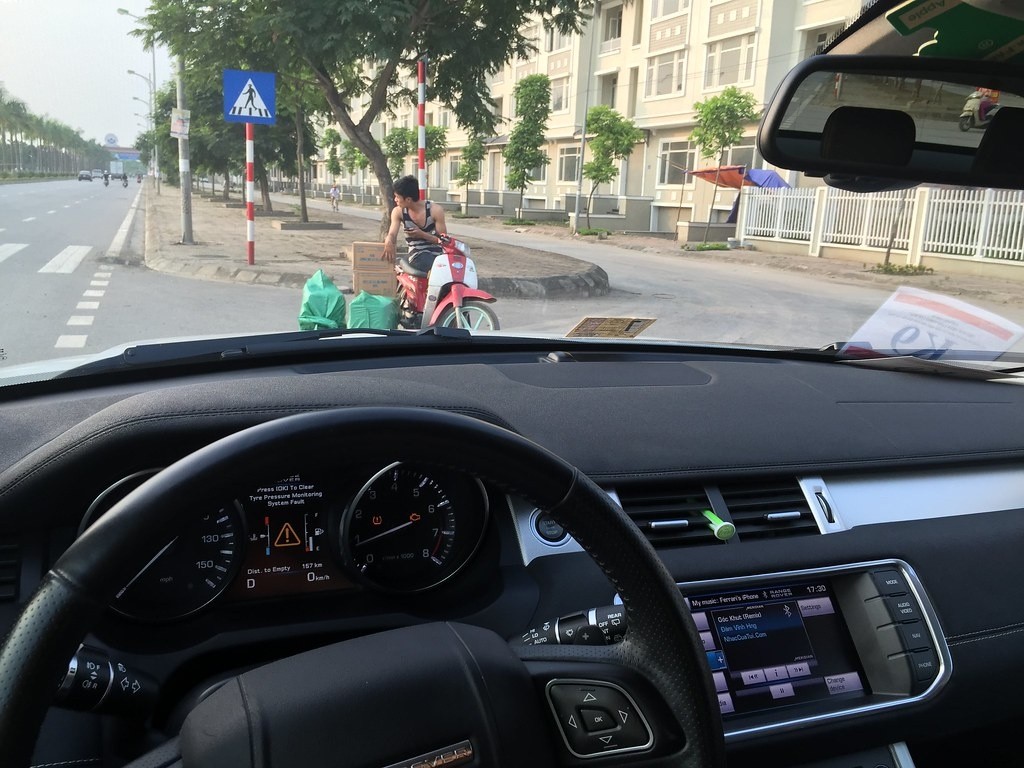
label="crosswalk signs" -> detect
[223,69,276,126]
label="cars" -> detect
[90,169,104,180]
[78,170,92,182]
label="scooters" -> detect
[122,177,129,188]
[398,227,501,332]
[957,90,1004,133]
[104,176,109,187]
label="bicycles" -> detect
[329,195,340,211]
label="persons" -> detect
[380,175,449,272]
[330,184,339,210]
[102,169,143,184]
[975,86,1000,120]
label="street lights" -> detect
[127,68,156,189]
[116,8,161,195]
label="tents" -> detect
[689,162,793,224]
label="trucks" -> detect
[110,161,123,181]
